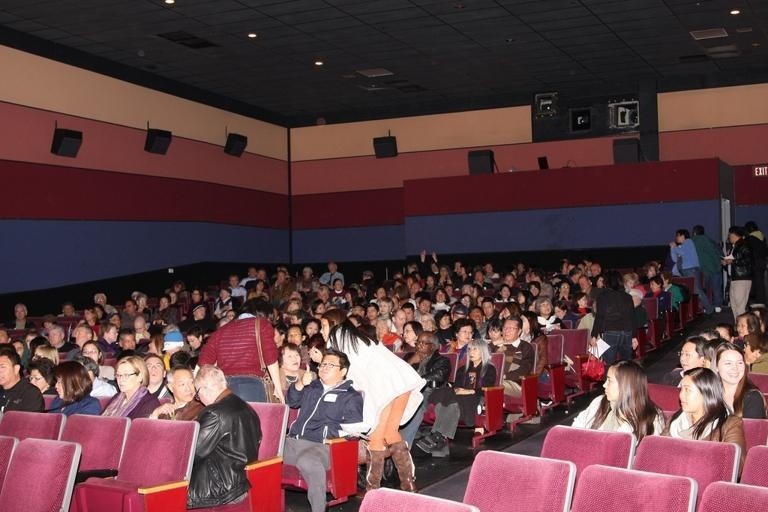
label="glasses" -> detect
[418,340,435,345]
[459,330,476,333]
[115,373,137,378]
[318,362,347,368]
[503,326,521,330]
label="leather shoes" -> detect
[415,431,447,453]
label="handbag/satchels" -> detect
[265,377,279,403]
[582,354,605,382]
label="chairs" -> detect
[2,435,21,493]
[738,443,767,489]
[539,423,635,499]
[697,482,767,512]
[568,462,699,512]
[629,434,742,511]
[60,413,132,511]
[2,438,82,511]
[2,275,767,450]
[279,435,360,508]
[241,401,290,512]
[357,488,481,511]
[462,451,578,512]
[75,418,200,511]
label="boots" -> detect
[354,440,417,499]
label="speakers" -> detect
[224,133,247,156]
[373,137,396,158]
[50,129,83,158]
[144,129,172,155]
[613,138,639,163]
[467,150,494,174]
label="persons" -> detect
[0,218,767,512]
